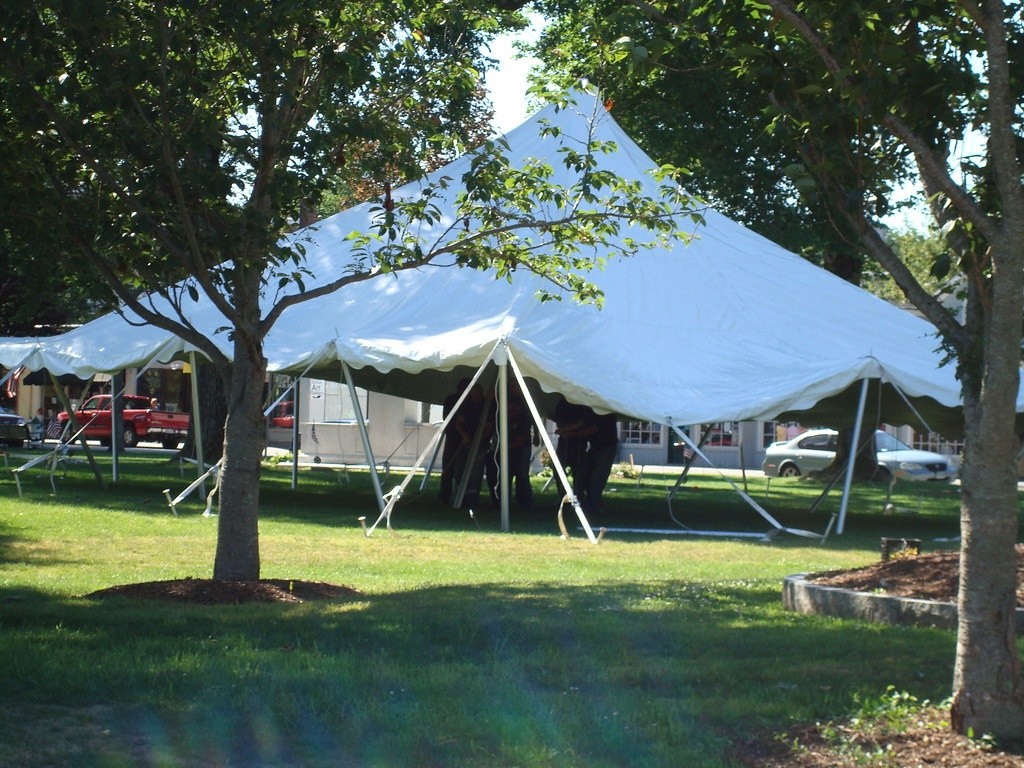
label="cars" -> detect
[761,428,957,485]
[0,407,32,447]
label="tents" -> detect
[0,87,1023,534]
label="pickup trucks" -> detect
[273,401,294,428]
[56,394,191,450]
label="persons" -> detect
[436,370,620,517]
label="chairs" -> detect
[24,421,44,451]
